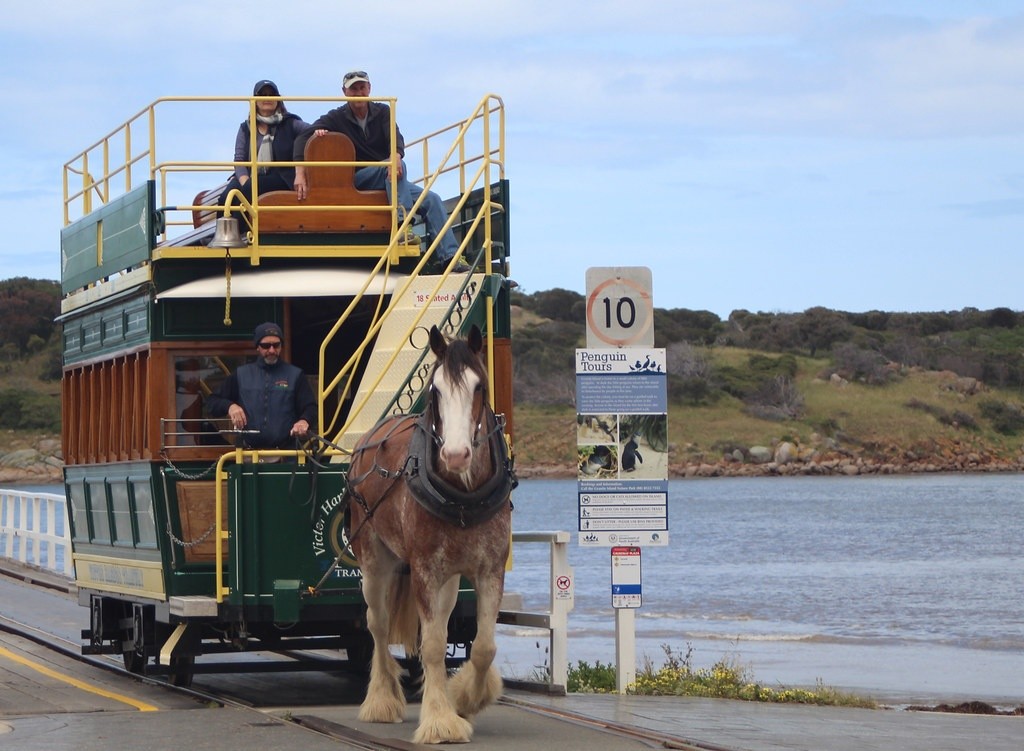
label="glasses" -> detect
[259,343,281,349]
[344,72,368,79]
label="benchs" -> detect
[154,131,393,247]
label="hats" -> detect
[253,322,284,347]
[342,71,370,88]
[254,80,278,96]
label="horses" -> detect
[348,324,510,744]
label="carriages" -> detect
[57,94,517,745]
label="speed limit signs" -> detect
[584,266,656,347]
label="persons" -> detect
[293,71,479,272]
[216,79,328,235]
[207,323,316,464]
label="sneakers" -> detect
[400,226,422,246]
[445,256,479,273]
